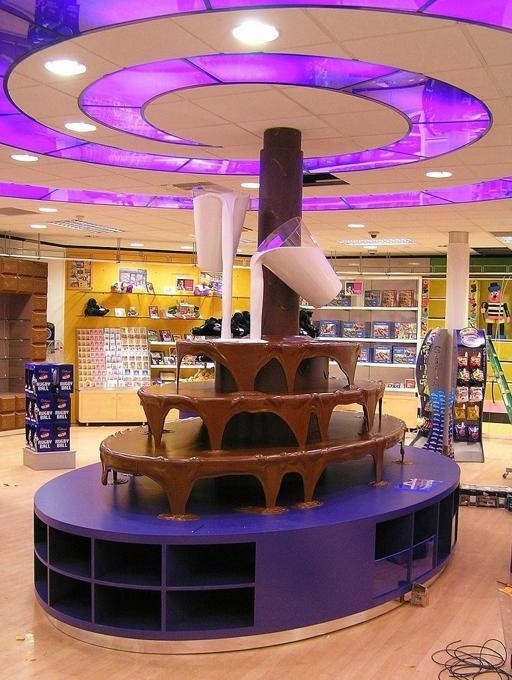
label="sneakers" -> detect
[192,317,222,335]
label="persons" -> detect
[480,282,511,339]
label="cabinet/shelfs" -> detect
[0,238,512,434]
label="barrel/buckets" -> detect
[257,216,343,310]
[192,186,250,272]
